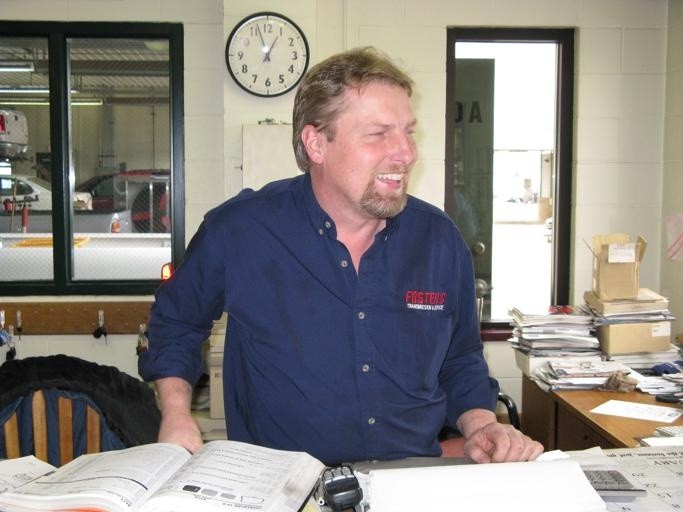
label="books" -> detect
[0,439,325,512]
[508,289,683,391]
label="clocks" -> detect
[224,11,310,99]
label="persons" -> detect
[138,45,545,463]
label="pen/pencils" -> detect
[317,481,326,505]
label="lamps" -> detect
[1,49,35,73]
[1,90,104,106]
[0,73,81,94]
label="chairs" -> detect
[0,355,160,470]
[439,393,520,459]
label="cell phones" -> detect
[321,464,364,512]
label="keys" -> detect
[16,327,23,341]
[92,327,108,347]
[0,322,15,360]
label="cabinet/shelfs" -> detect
[523,345,683,452]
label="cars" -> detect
[0,174,92,214]
[75,170,170,211]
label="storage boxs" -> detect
[602,322,673,358]
[584,232,647,301]
